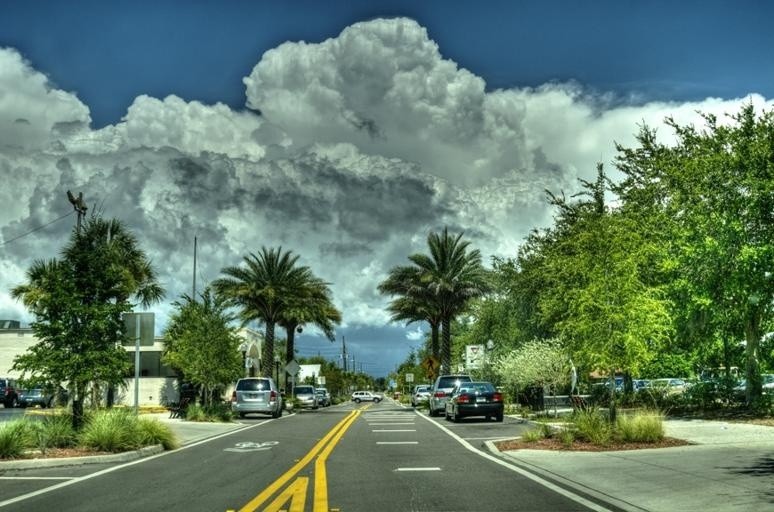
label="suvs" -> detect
[351,391,383,403]
[693,364,740,391]
[231,378,285,419]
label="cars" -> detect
[295,384,331,410]
[411,373,510,424]
[0,380,69,409]
[733,373,774,393]
[602,375,698,399]
